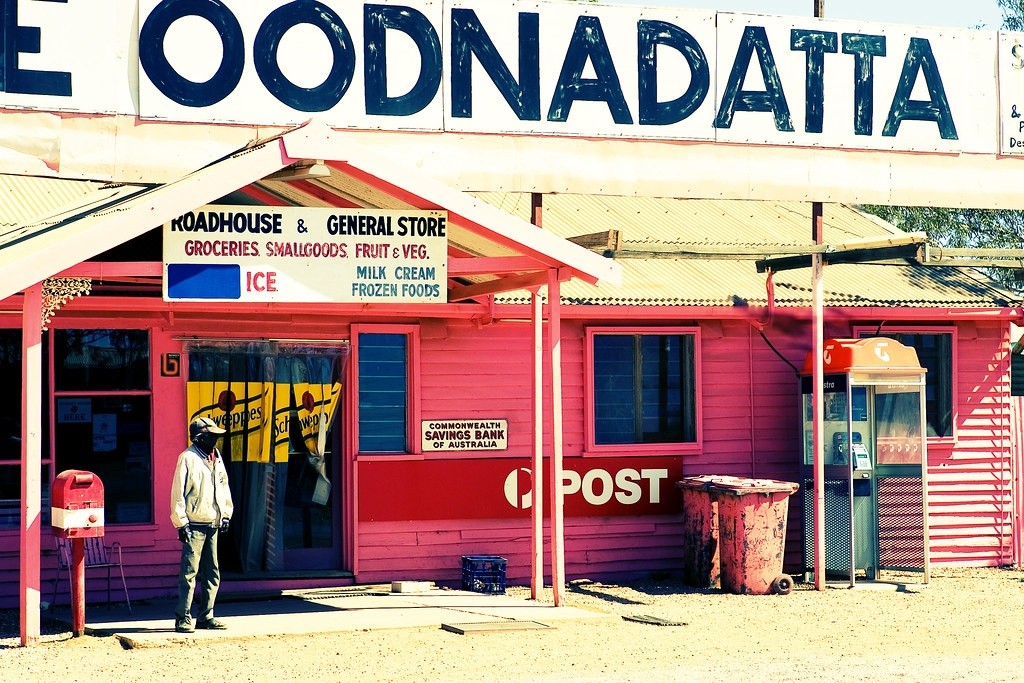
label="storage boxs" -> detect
[461,554,507,595]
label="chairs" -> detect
[50,537,133,618]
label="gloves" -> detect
[179,527,196,543]
[220,520,230,534]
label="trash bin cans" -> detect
[709,479,800,595]
[675,474,746,590]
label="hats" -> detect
[190,417,226,437]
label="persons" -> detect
[170,417,234,633]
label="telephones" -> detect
[839,442,872,471]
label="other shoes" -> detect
[194,619,227,629]
[175,623,195,633]
